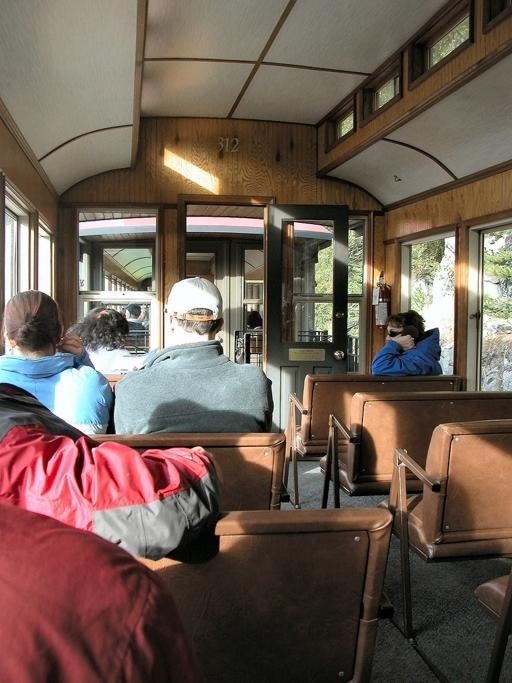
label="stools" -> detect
[248,334,351,368]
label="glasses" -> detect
[387,331,402,337]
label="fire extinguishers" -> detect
[374,271,392,330]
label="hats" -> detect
[121,304,141,316]
[246,311,263,328]
[166,276,222,323]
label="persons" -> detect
[125,304,146,330]
[246,310,263,331]
[112,276,274,436]
[0,503,206,682]
[371,309,443,378]
[0,290,113,435]
[0,383,223,561]
[58,307,156,375]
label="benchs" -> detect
[120,329,149,353]
[0,374,512,679]
